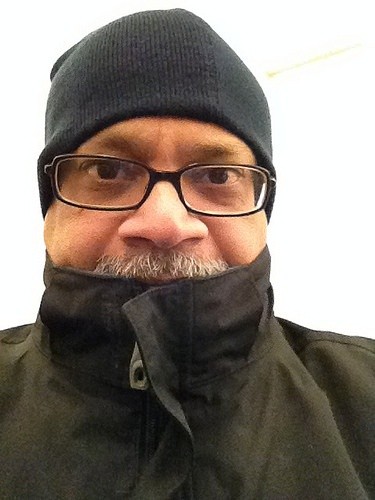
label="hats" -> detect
[38,8,277,221]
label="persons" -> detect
[1,8,375,499]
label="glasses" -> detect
[43,154,273,218]
